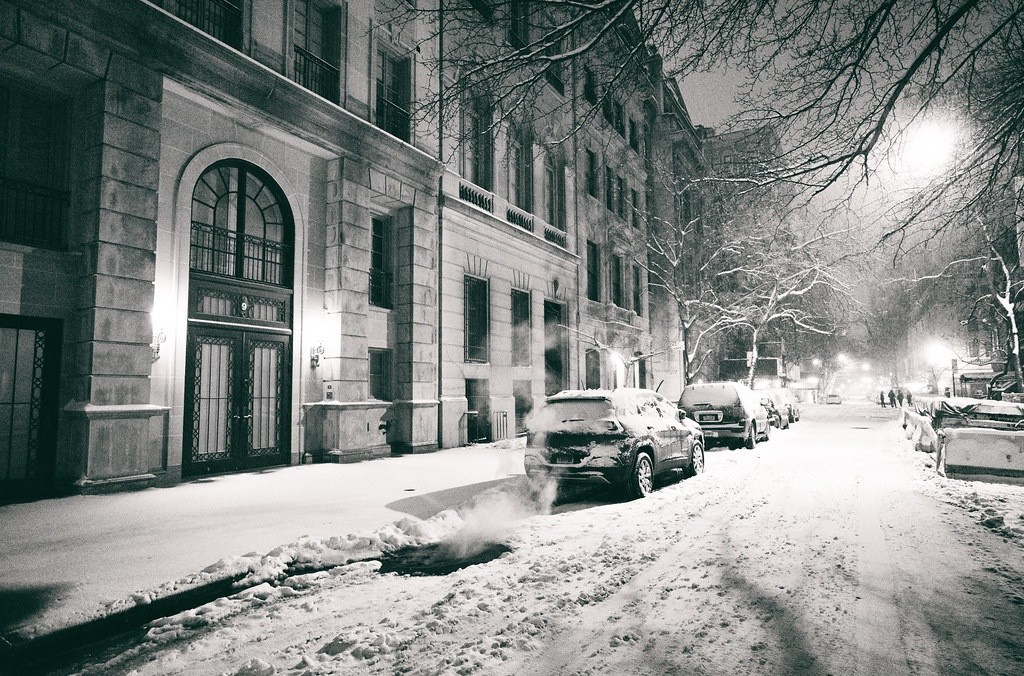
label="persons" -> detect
[880,390,912,407]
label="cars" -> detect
[826,393,842,404]
[750,385,800,431]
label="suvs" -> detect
[524,388,706,499]
[677,378,771,450]
